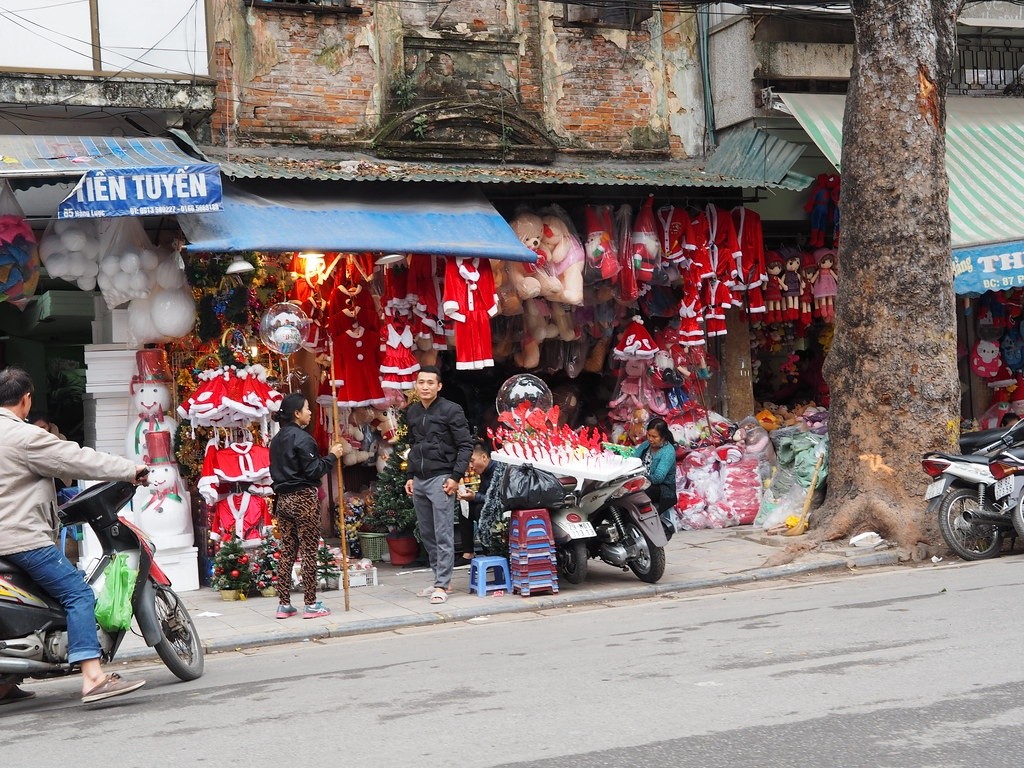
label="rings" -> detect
[452,491,455,495]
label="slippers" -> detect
[417,587,454,597]
[430,592,448,604]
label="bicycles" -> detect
[549,460,675,584]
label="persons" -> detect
[402,365,473,603]
[454,440,499,566]
[31,416,74,490]
[1000,413,1020,427]
[268,393,343,620]
[0,369,150,703]
[631,418,678,515]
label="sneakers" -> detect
[302,601,331,618]
[0,683,36,704]
[276,604,297,618]
[82,673,146,702]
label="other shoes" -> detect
[468,567,494,574]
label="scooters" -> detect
[0,465,205,684]
[919,412,1024,562]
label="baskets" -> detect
[357,532,389,561]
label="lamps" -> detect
[372,253,405,265]
[297,251,326,258]
[226,255,255,274]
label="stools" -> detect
[469,508,559,596]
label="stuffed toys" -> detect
[494,196,664,378]
[323,407,400,473]
[804,171,839,248]
[970,288,1024,420]
[126,350,195,533]
[753,247,836,384]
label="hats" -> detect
[611,317,660,361]
[176,364,283,429]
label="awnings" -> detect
[770,91,1024,293]
[0,132,224,219]
[173,187,537,264]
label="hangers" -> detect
[232,482,244,510]
[660,195,742,220]
[232,426,251,451]
[345,274,360,335]
[391,310,406,331]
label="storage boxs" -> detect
[119,547,201,593]
[325,567,378,590]
[358,531,389,561]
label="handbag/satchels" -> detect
[94,553,132,632]
[502,463,564,509]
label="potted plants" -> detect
[210,526,251,601]
[317,530,334,590]
[367,390,418,566]
[252,527,282,597]
